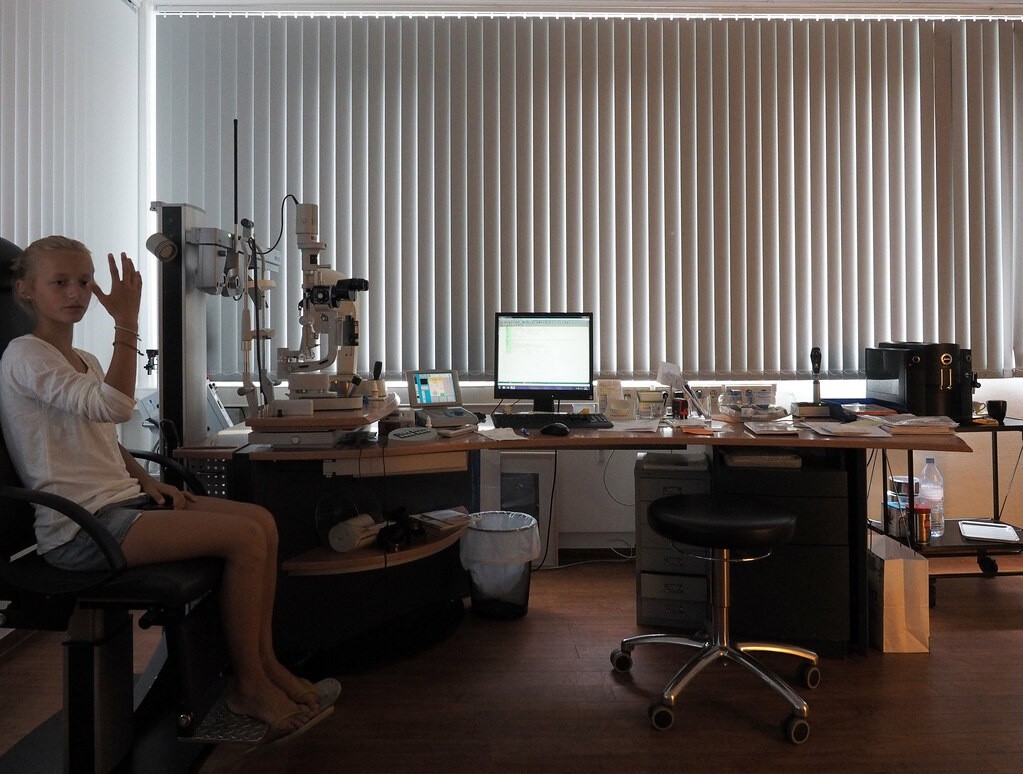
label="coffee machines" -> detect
[862,343,983,428]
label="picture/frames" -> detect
[498,449,561,572]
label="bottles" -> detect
[918,457,945,538]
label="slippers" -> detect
[241,678,342,759]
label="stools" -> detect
[607,489,824,748]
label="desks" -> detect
[171,387,977,669]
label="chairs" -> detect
[0,236,235,774]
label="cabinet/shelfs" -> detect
[867,413,1023,613]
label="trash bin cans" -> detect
[458,510,541,622]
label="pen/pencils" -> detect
[520,427,530,436]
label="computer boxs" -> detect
[879,341,960,426]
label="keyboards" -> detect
[491,413,613,430]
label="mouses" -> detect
[540,422,569,436]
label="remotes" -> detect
[438,423,478,438]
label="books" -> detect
[744,400,959,442]
[409,508,474,530]
[664,418,707,430]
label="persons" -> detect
[3,232,334,733]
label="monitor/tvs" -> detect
[494,312,593,400]
[865,347,911,410]
[406,370,463,407]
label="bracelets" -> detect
[114,325,142,339]
[111,340,144,355]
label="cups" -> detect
[986,400,1007,424]
[971,400,986,415]
